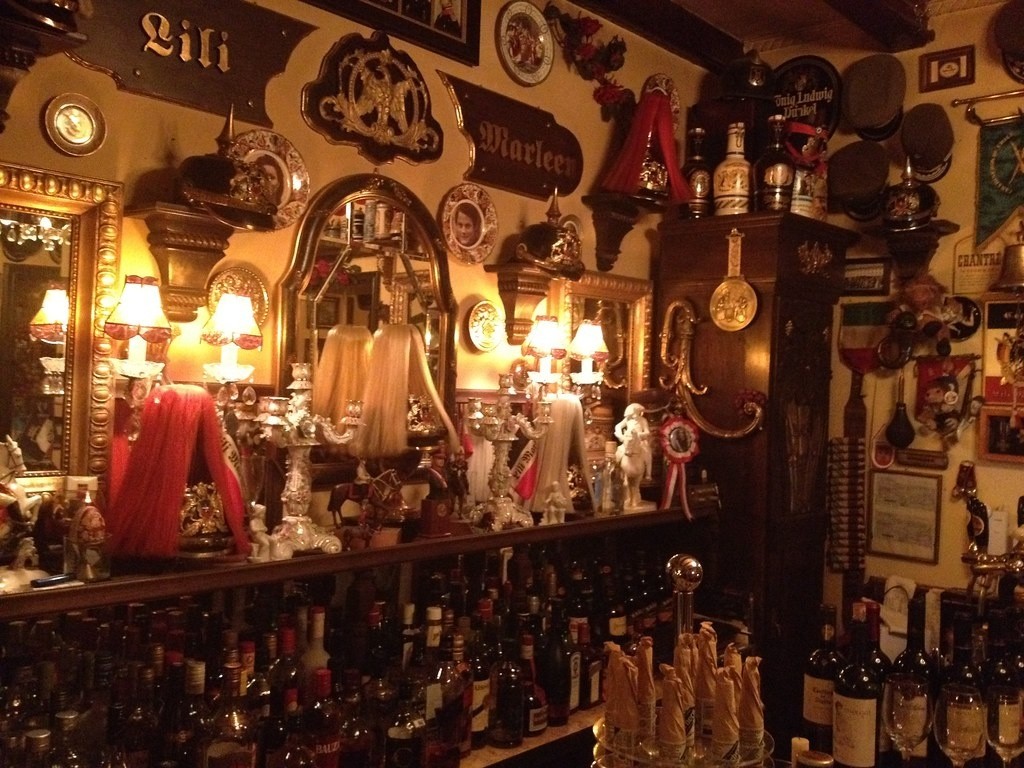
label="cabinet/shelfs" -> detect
[0,502,722,768]
[652,212,861,761]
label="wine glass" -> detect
[988,694,1024,768]
[935,684,988,768]
[884,673,934,768]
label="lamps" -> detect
[29,277,68,397]
[520,314,567,384]
[569,318,610,386]
[199,294,263,385]
[104,273,173,381]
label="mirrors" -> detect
[558,271,655,466]
[0,160,127,520]
[273,167,460,485]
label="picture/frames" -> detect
[306,297,341,330]
[977,405,1024,465]
[299,0,482,67]
[839,257,893,297]
[439,183,499,266]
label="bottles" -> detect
[752,114,795,211]
[0,541,765,768]
[801,598,1024,768]
[680,129,710,220]
[713,122,752,215]
